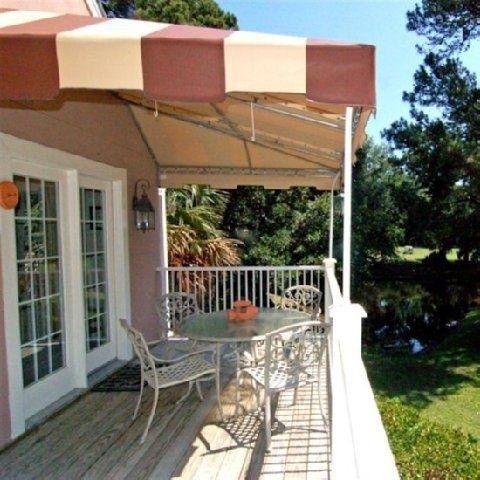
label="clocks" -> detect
[0,182,19,209]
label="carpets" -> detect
[91,361,170,392]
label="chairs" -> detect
[118,285,334,452]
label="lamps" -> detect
[133,178,155,234]
